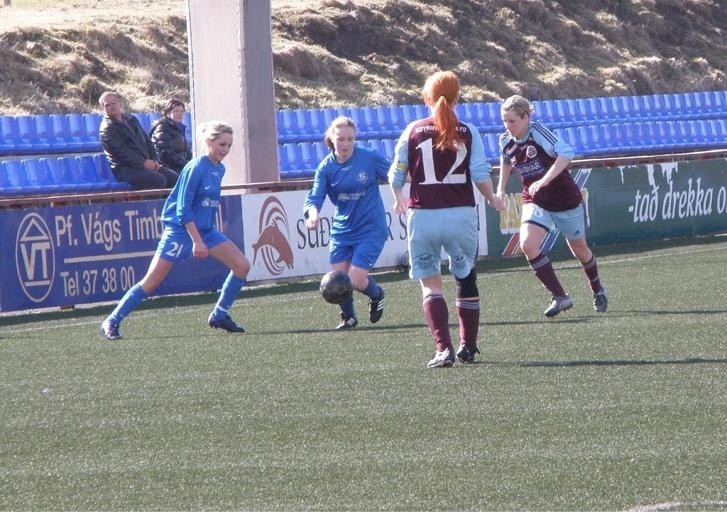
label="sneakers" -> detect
[591,288,608,312]
[367,287,384,323]
[428,347,454,367]
[207,312,245,332]
[455,344,480,363]
[99,317,122,340]
[335,313,358,330]
[544,291,574,317]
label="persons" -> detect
[149,99,195,173]
[494,93,609,316]
[98,118,253,342]
[300,115,413,332]
[383,70,506,370]
[97,91,181,200]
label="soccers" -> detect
[320,270,353,303]
